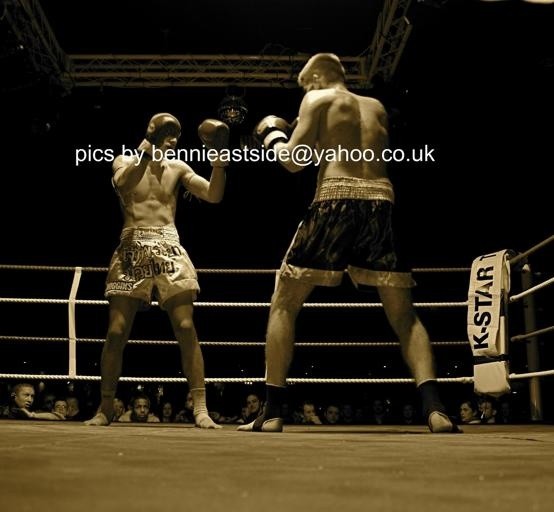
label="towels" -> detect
[465,246,518,397]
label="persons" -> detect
[82,112,229,429]
[0,377,527,424]
[237,52,464,434]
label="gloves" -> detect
[198,119,230,168]
[136,112,182,160]
[252,115,291,150]
